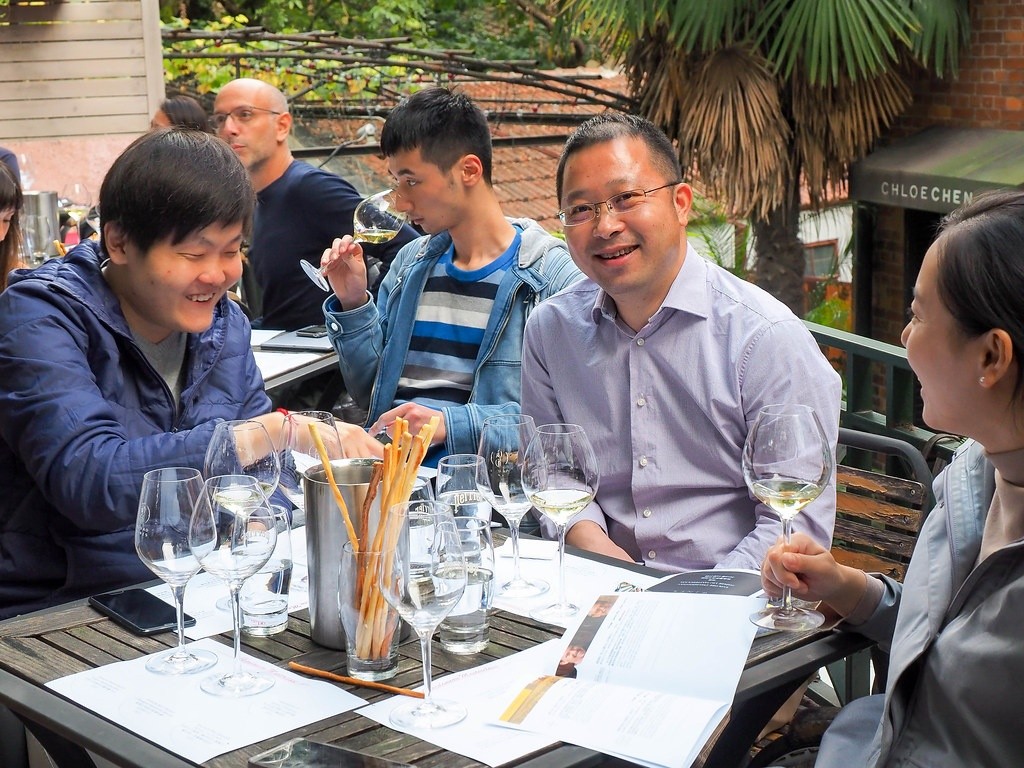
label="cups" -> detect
[17,154,37,190]
[338,539,406,681]
[19,190,61,258]
[436,518,495,654]
[409,476,434,525]
[435,454,494,530]
[234,506,293,637]
[304,457,412,650]
[20,216,50,269]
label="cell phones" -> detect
[296,326,328,338]
[88,588,196,637]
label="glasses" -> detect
[556,181,684,227]
[208,105,280,130]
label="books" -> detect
[487,592,771,768]
[644,568,769,597]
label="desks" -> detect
[0,517,876,768]
[248,327,342,396]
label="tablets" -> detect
[260,329,335,353]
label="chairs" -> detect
[740,428,937,768]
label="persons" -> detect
[519,113,842,572]
[320,84,588,471]
[0,160,29,294]
[560,642,591,678]
[214,78,421,333]
[0,127,388,623]
[0,147,21,186]
[149,95,213,134]
[759,192,1024,766]
[587,600,613,617]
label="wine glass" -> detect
[135,466,219,676]
[61,183,91,245]
[299,188,408,293]
[278,411,345,584]
[380,500,468,730]
[188,474,275,698]
[475,413,550,598]
[521,423,600,621]
[203,421,282,613]
[741,403,832,633]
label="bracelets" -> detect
[277,408,292,445]
